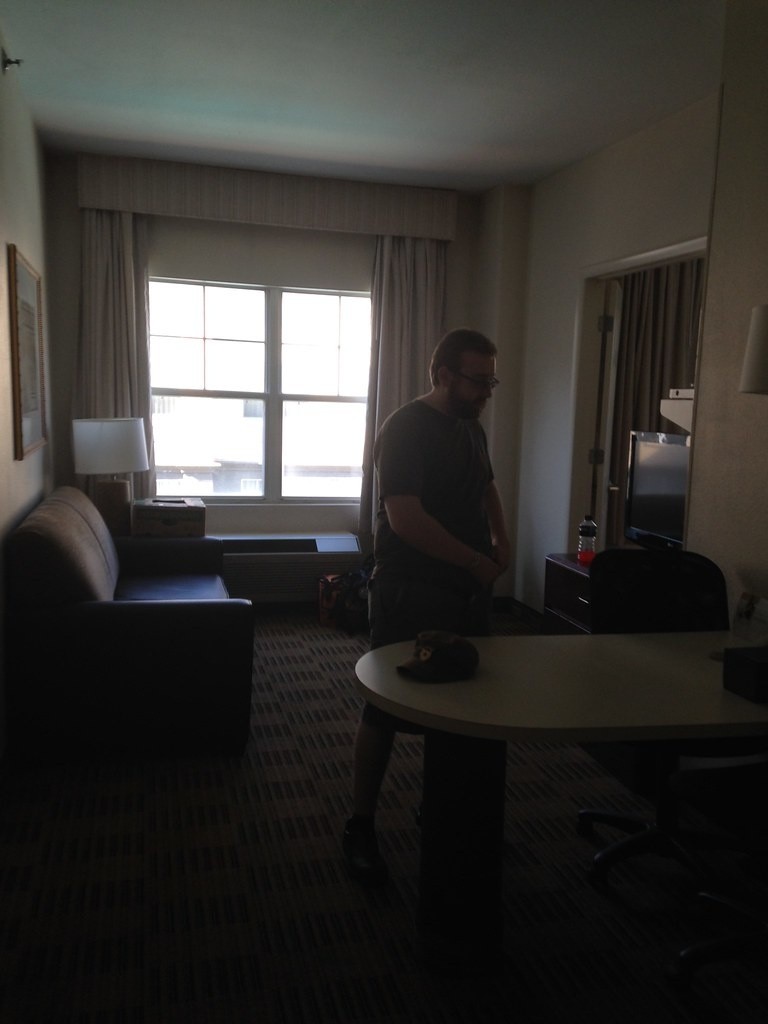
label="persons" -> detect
[337,326,517,894]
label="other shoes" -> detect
[341,814,386,889]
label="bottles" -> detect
[578,515,597,561]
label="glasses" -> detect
[449,371,500,390]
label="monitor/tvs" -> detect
[623,430,691,553]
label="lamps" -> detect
[70,414,151,532]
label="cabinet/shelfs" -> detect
[541,551,602,636]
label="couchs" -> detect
[2,486,258,762]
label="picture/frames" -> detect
[3,239,53,462]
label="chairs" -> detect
[590,546,735,634]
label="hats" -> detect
[394,629,478,684]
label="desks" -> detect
[342,628,768,930]
